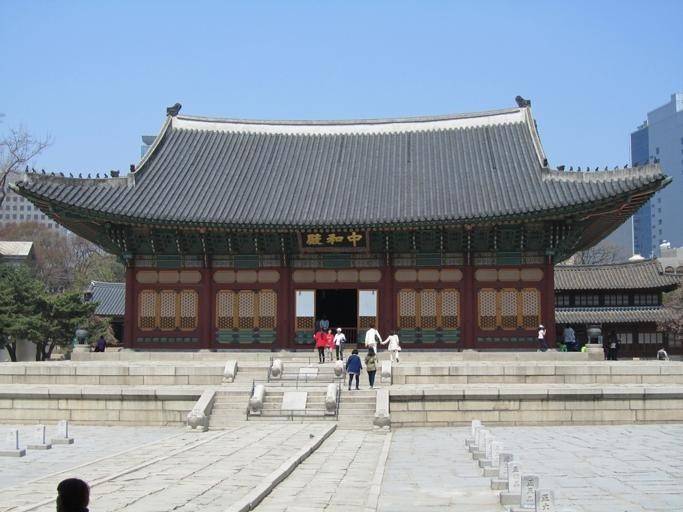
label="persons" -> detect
[313,315,346,363]
[562,323,577,352]
[346,349,362,389]
[382,329,401,363]
[97,335,106,351]
[603,338,618,361]
[657,346,670,360]
[364,346,378,389]
[537,324,551,352]
[56,479,89,511]
[364,323,382,356]
